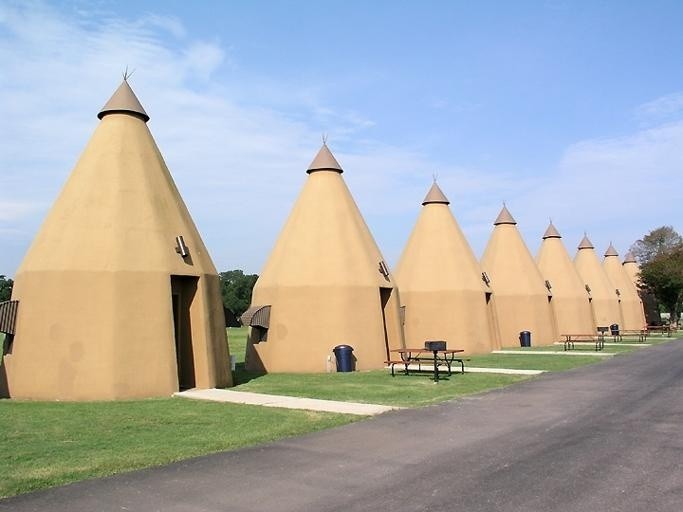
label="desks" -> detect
[390,349,464,376]
[646,325,670,336]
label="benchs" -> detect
[407,358,470,374]
[384,361,448,377]
[559,329,673,350]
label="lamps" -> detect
[175,235,190,258]
[482,272,490,283]
[616,288,620,295]
[584,284,591,294]
[545,280,552,289]
[378,261,390,276]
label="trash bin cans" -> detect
[610,325,617,335]
[333,344,353,371]
[520,331,530,347]
